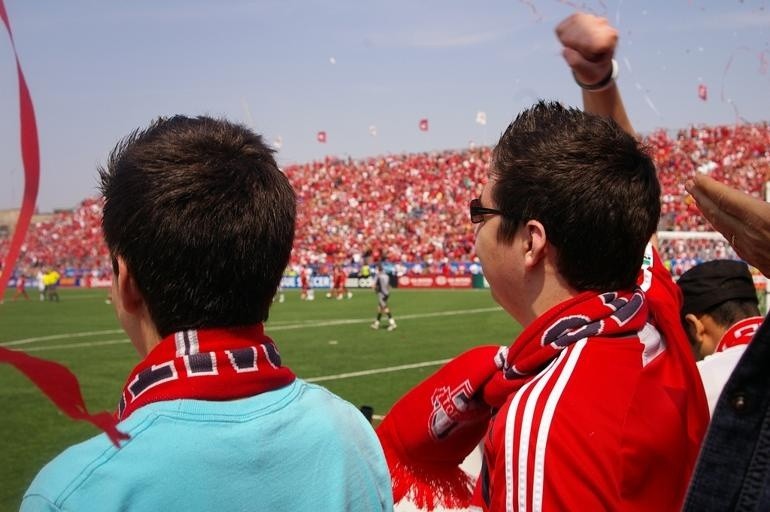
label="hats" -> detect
[680,260,762,317]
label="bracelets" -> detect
[572,58,620,92]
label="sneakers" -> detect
[299,293,352,301]
[370,323,378,330]
[387,325,396,331]
[278,296,284,303]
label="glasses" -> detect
[469,198,532,225]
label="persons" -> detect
[678,259,766,418]
[272,144,496,305]
[370,13,714,511]
[681,173,770,511]
[1,195,114,304]
[372,265,397,332]
[639,120,770,275]
[18,114,394,511]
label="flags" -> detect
[317,131,326,143]
[699,86,706,100]
[476,112,486,124]
[419,119,428,131]
